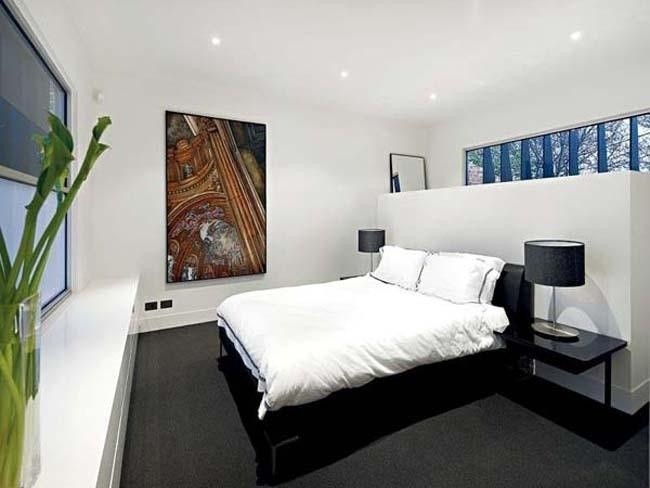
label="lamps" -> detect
[358,229,386,272]
[524,239,585,338]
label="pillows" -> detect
[370,246,506,304]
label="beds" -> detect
[218,263,533,485]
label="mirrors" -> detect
[390,153,427,193]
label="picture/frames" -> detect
[165,110,266,284]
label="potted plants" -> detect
[1,112,112,487]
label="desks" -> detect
[493,317,628,416]
